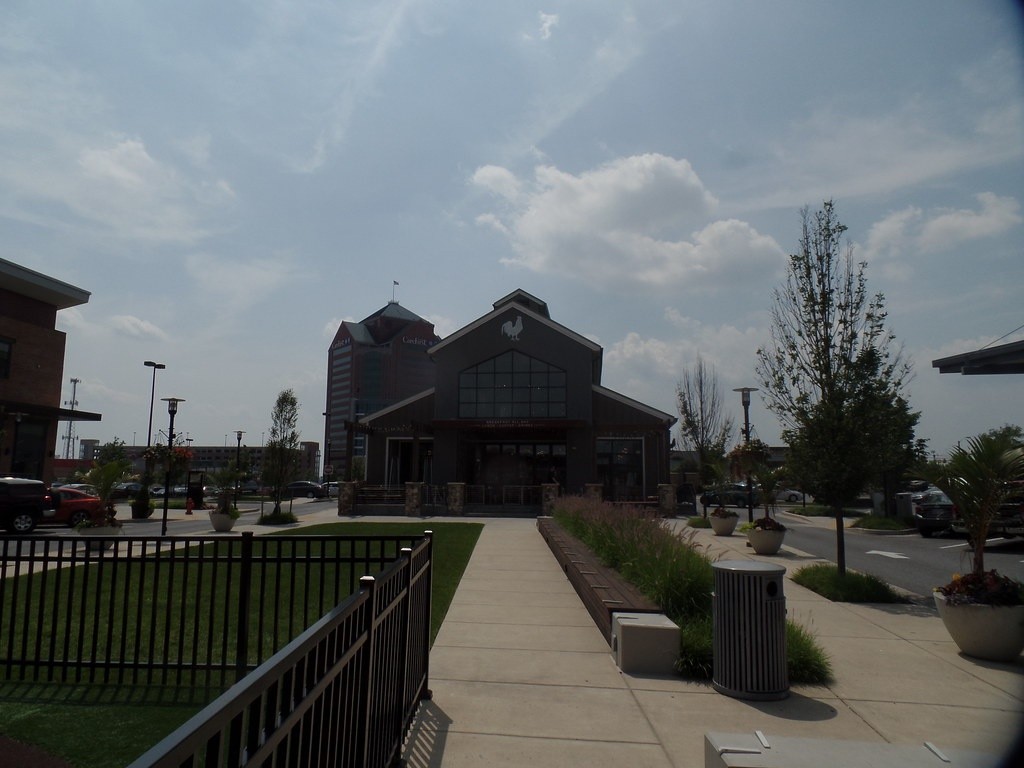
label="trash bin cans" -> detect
[706,561,791,702]
[895,493,913,518]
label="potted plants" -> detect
[902,425,1024,659]
[704,457,740,536]
[73,450,124,549]
[205,458,250,532]
[744,458,788,555]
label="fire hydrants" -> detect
[185,498,195,514]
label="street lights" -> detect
[733,388,760,546]
[323,413,330,498]
[143,362,166,469]
[65,379,81,459]
[160,397,187,535]
[232,431,247,509]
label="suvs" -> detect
[968,480,1024,548]
[0,477,56,535]
[238,480,258,495]
[914,490,971,539]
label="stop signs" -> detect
[324,465,334,474]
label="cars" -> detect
[149,487,186,496]
[752,483,803,502]
[698,483,760,508]
[203,486,235,496]
[906,479,937,491]
[52,487,105,527]
[272,481,325,499]
[321,481,340,497]
[59,484,99,497]
[116,483,155,498]
